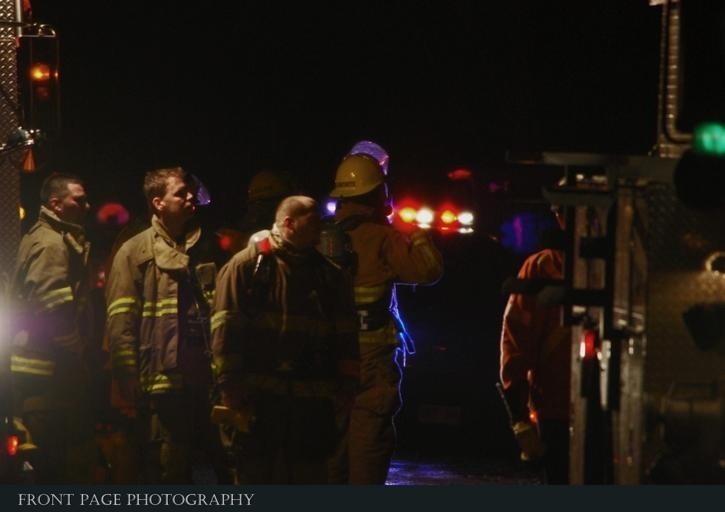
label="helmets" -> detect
[329,153,385,198]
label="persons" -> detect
[497,204,609,485]
